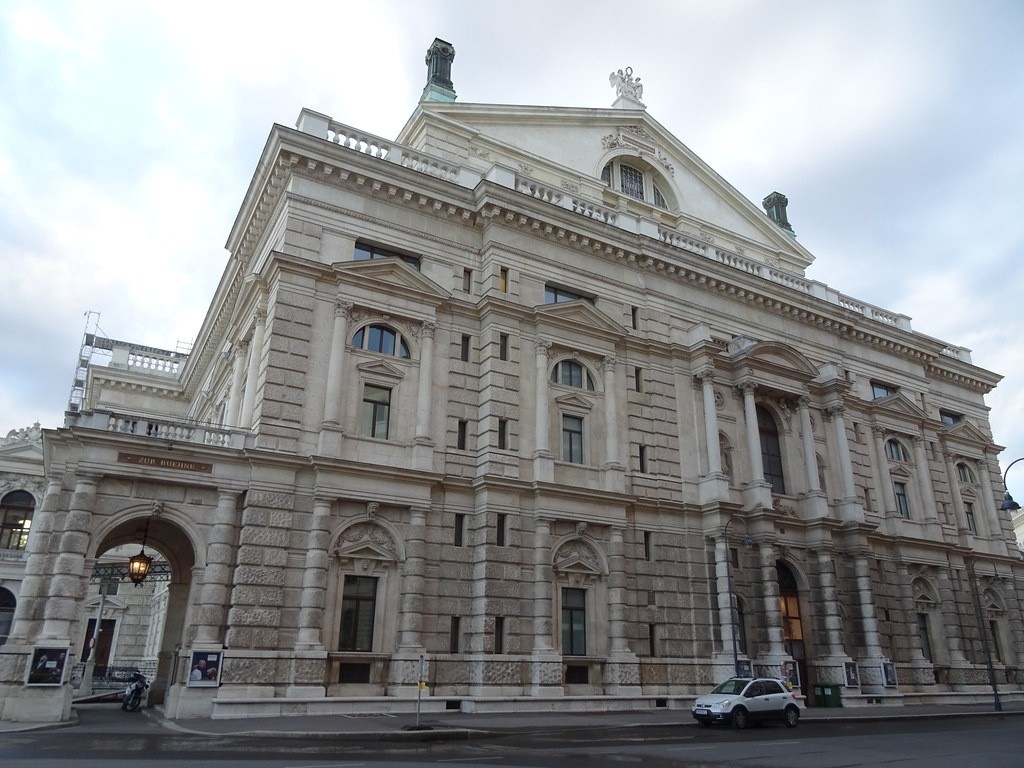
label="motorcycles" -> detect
[121,673,149,712]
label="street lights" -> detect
[724,516,753,676]
[972,557,1002,711]
[1000,458,1024,510]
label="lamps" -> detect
[128,518,153,588]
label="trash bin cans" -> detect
[812,682,845,708]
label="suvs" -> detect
[691,677,801,729]
